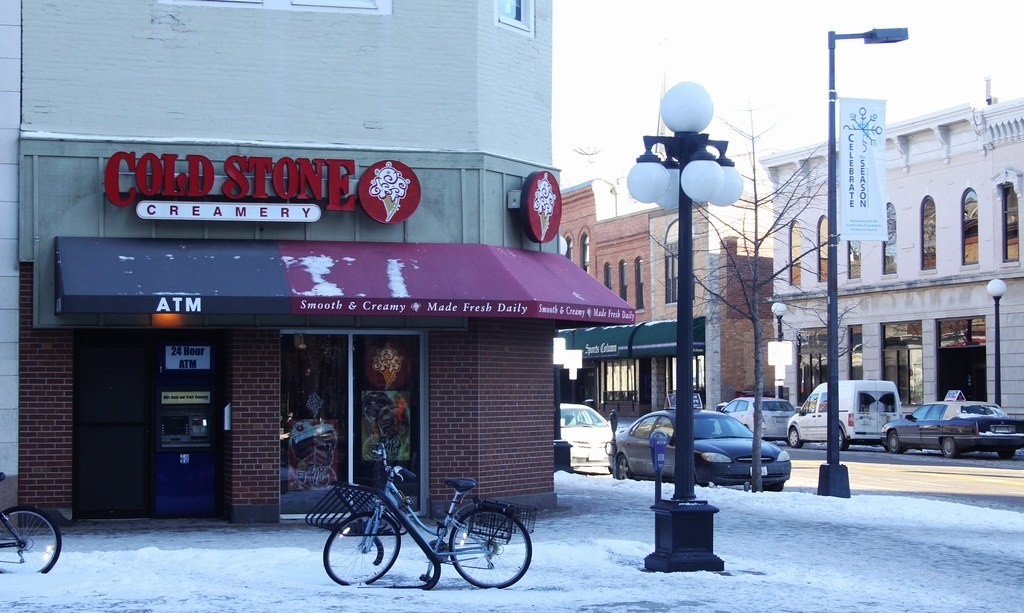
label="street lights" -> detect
[771,302,789,399]
[626,80,744,573]
[987,279,1009,408]
[816,27,911,498]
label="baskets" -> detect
[468,497,513,545]
[475,499,538,534]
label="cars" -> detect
[718,397,795,444]
[881,390,1024,461]
[559,403,614,470]
[607,409,791,492]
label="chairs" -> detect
[695,419,715,436]
[564,415,576,426]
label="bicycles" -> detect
[323,435,536,591]
[0,472,62,576]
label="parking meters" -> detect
[610,410,619,479]
[649,428,667,504]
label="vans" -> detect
[786,379,902,450]
